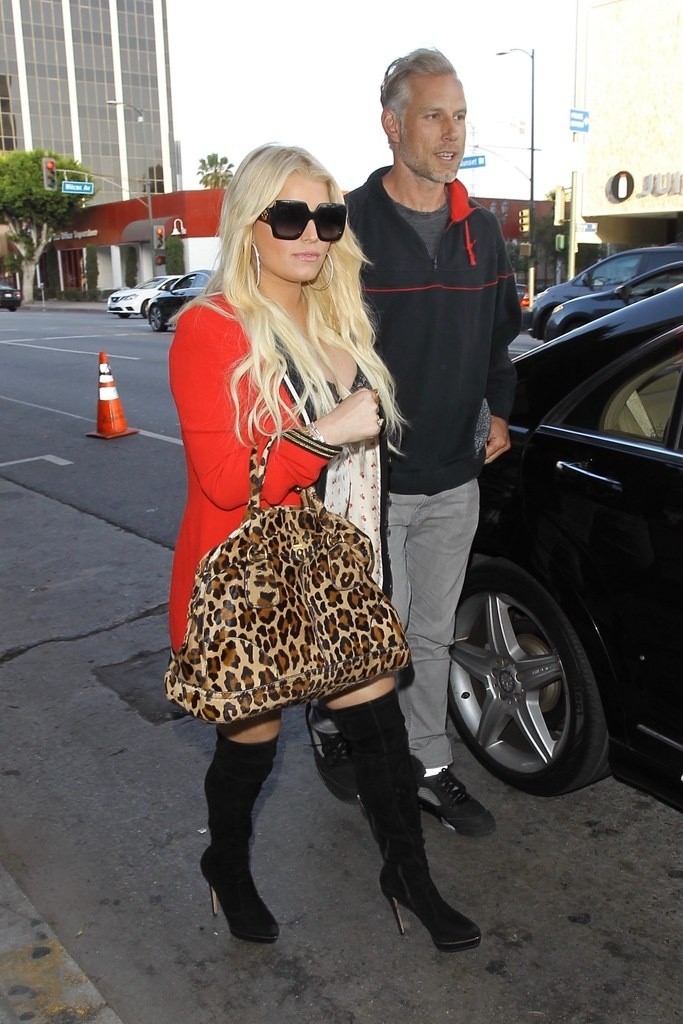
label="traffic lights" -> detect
[41,158,58,192]
[155,255,162,266]
[153,225,165,249]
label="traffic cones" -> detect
[85,351,139,440]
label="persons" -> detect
[168,146,481,953]
[306,49,523,837]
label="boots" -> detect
[200,736,280,943]
[328,689,482,951]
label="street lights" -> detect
[496,47,537,297]
[105,100,158,278]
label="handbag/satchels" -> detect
[165,487,410,724]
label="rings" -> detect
[377,415,379,421]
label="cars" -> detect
[527,245,683,340]
[107,274,195,318]
[446,283,683,798]
[543,260,683,345]
[146,269,217,332]
[515,283,529,317]
[0,282,22,312]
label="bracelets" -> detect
[302,422,326,444]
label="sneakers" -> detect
[305,702,360,803]
[417,769,495,838]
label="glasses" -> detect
[256,200,347,242]
